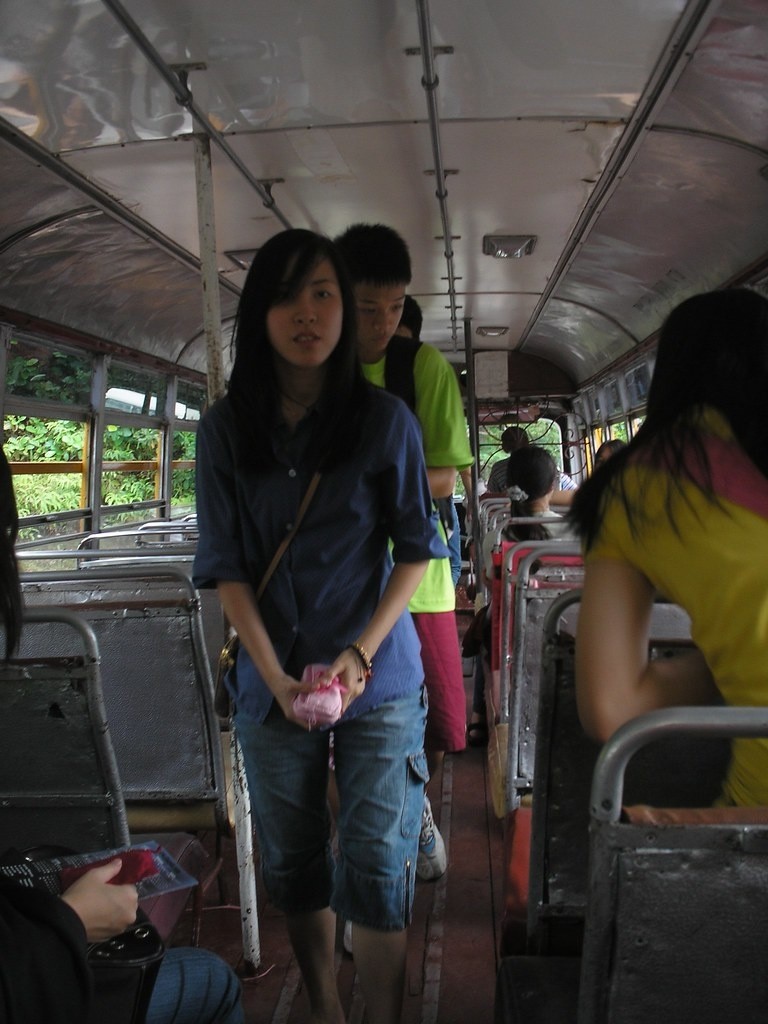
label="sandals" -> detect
[468,723,486,742]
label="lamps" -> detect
[483,233,537,258]
[224,247,258,272]
[478,325,509,337]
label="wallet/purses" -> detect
[293,663,348,732]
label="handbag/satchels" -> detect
[0,843,166,968]
[213,635,241,717]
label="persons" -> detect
[193,227,452,1024]
[563,288,768,812]
[0,450,247,1024]
[331,223,634,951]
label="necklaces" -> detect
[280,389,309,411]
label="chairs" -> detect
[467,491,767,1024]
[1,511,272,977]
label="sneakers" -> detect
[343,920,353,953]
[417,795,447,881]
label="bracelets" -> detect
[352,642,374,669]
[346,644,371,682]
